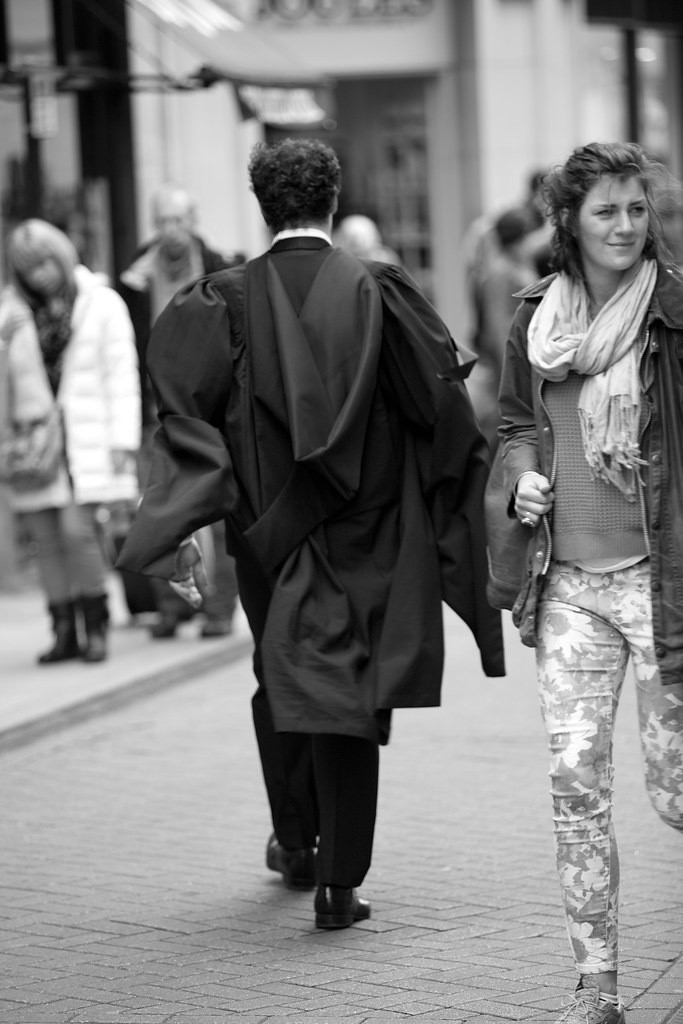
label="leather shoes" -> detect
[266,831,316,890]
[316,886,376,930]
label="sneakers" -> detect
[554,974,627,1024]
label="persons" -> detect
[114,139,504,931]
[495,143,683,1024]
[0,219,141,661]
[114,165,549,638]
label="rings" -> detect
[526,511,530,516]
[521,518,533,526]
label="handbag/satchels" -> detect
[482,442,537,613]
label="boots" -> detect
[83,596,110,661]
[38,602,79,664]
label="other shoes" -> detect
[151,612,195,636]
[201,622,231,637]
